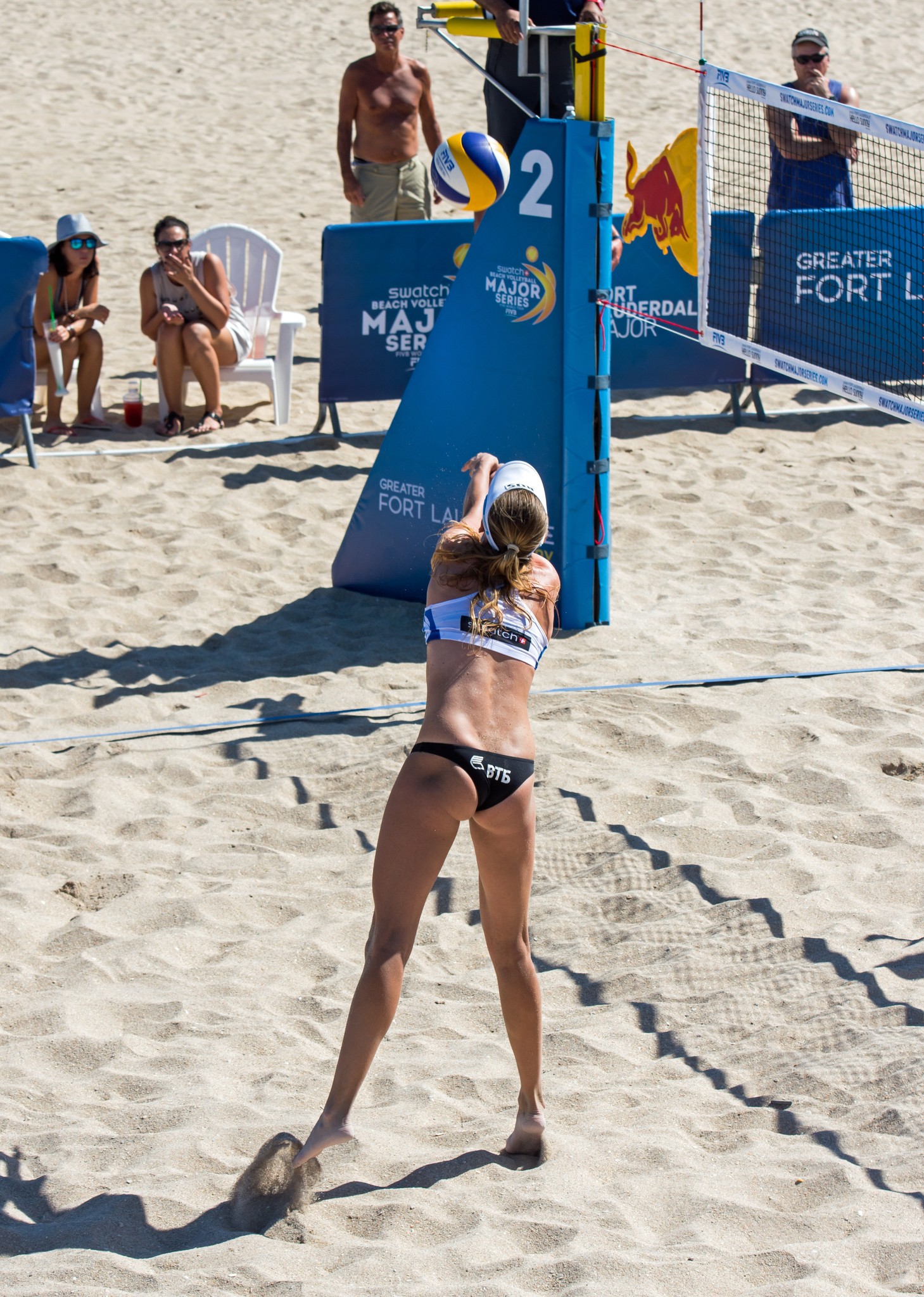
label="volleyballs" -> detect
[430,131,510,212]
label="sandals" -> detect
[155,410,185,437]
[188,409,225,437]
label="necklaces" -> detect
[63,276,82,312]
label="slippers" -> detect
[45,426,77,437]
[73,416,114,431]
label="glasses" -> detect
[155,238,189,253]
[70,237,97,250]
[793,53,826,64]
[369,25,401,35]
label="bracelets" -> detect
[66,309,80,322]
[584,0,604,11]
[66,325,76,337]
[613,235,623,244]
[828,96,835,100]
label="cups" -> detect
[42,320,69,396]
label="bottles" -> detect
[123,381,144,427]
[562,106,576,120]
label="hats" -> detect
[792,28,830,49]
[482,459,549,558]
[47,212,109,251]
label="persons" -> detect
[34,214,112,437]
[287,454,560,1172]
[472,0,622,271]
[141,216,252,437]
[764,28,859,209]
[337,2,445,223]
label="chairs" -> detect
[0,231,103,470]
[157,224,305,424]
